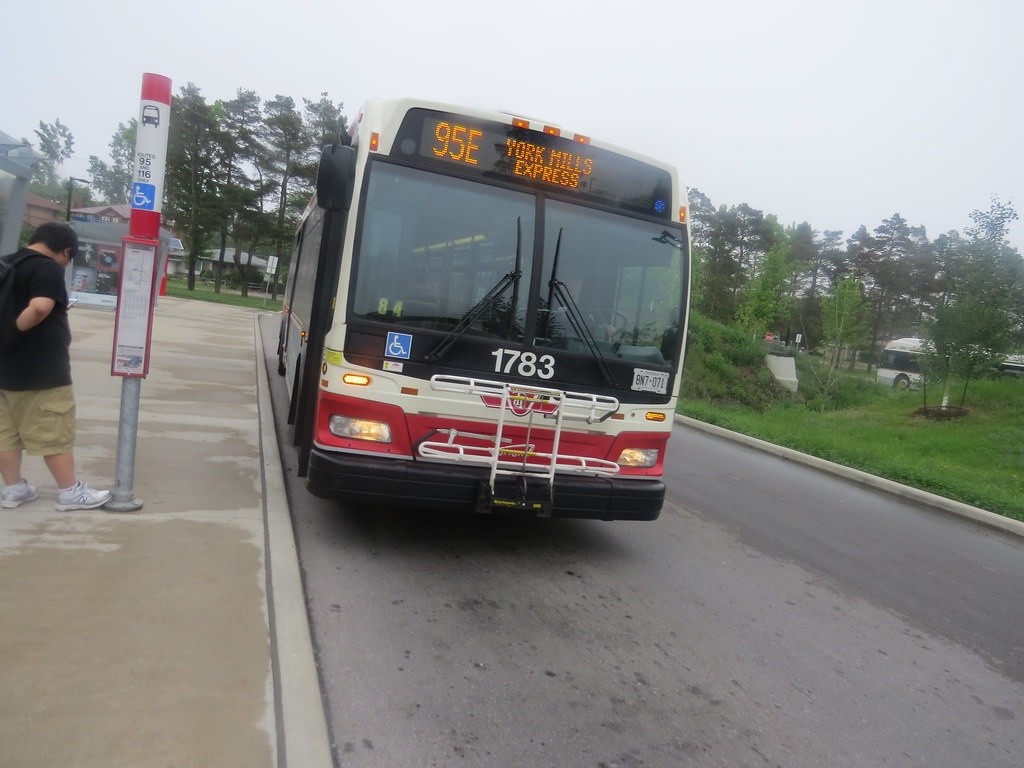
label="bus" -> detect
[276,95,693,522]
[876,337,1024,395]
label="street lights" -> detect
[67,176,90,225]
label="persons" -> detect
[766,331,779,342]
[0,222,113,511]
[800,346,805,351]
[781,339,785,346]
[562,272,617,340]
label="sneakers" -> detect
[54,481,112,511]
[0,479,39,509]
